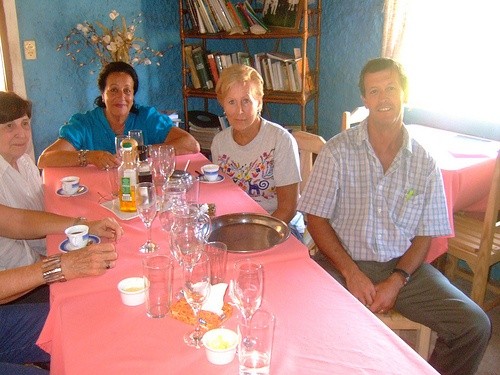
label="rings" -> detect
[381,310,385,313]
[105,261,110,269]
[105,165,109,168]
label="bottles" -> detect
[168,206,212,260]
[160,184,190,233]
[137,146,153,202]
[118,143,139,212]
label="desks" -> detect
[406,123,499,264]
[42,152,440,373]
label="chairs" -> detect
[292,130,326,250]
[342,105,500,360]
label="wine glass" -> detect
[135,146,204,300]
[135,183,159,254]
[181,253,210,348]
[231,260,262,358]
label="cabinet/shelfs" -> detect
[180,0,321,160]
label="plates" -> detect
[197,175,225,183]
[59,234,101,253]
[57,186,88,196]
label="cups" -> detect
[129,130,143,146]
[115,136,130,163]
[61,176,79,193]
[65,225,89,249]
[238,308,274,375]
[149,144,165,185]
[204,242,228,285]
[201,164,219,181]
[144,255,174,318]
[107,166,120,197]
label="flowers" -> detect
[57,7,174,78]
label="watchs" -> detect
[392,269,410,284]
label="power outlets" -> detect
[23,41,37,60]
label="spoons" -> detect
[195,171,204,178]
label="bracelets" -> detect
[73,217,87,224]
[78,149,90,167]
[40,254,65,284]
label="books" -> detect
[183,0,314,151]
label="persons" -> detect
[37,62,201,171]
[0,205,124,375]
[297,58,492,375]
[0,91,50,303]
[210,64,305,242]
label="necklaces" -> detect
[115,123,125,132]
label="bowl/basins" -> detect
[169,170,193,193]
[203,329,240,365]
[118,277,150,306]
